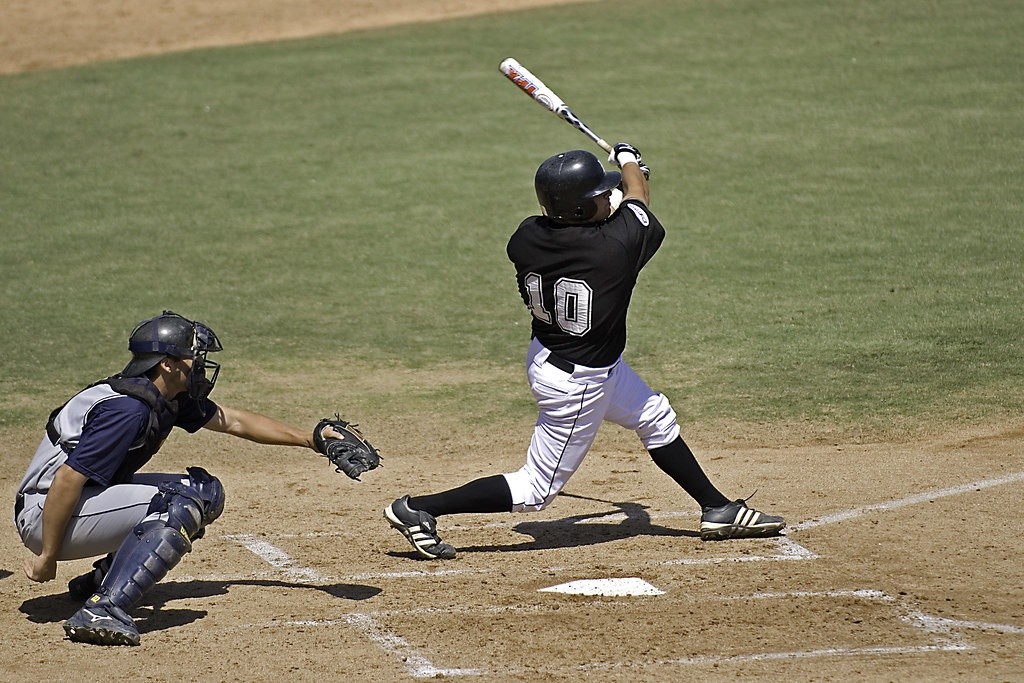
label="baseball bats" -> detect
[498,56,652,182]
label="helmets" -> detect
[535,150,621,219]
[119,310,195,378]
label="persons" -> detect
[13,309,380,646]
[382,143,789,559]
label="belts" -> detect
[546,354,613,376]
[13,498,25,519]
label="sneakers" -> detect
[61,605,141,645]
[383,494,457,558]
[69,571,95,603]
[699,489,786,540]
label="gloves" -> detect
[608,142,641,166]
[616,163,651,188]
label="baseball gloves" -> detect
[314,416,381,478]
